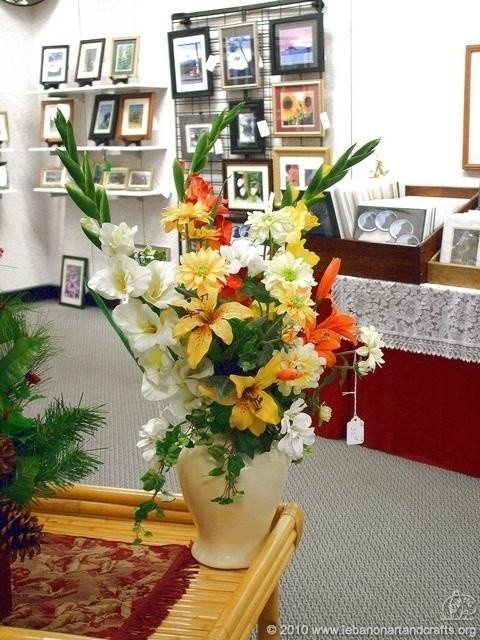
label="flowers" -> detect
[40,87,388,554]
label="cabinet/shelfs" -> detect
[0,146,20,197]
[26,80,171,205]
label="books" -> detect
[278,177,480,269]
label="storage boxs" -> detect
[422,241,480,288]
[296,179,480,287]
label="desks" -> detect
[0,481,310,639]
[312,268,479,482]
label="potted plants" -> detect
[0,264,110,623]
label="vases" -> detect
[166,411,295,575]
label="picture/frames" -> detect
[28,37,161,194]
[162,9,328,217]
[0,160,10,190]
[461,40,479,172]
[59,253,87,310]
[1,109,12,144]
